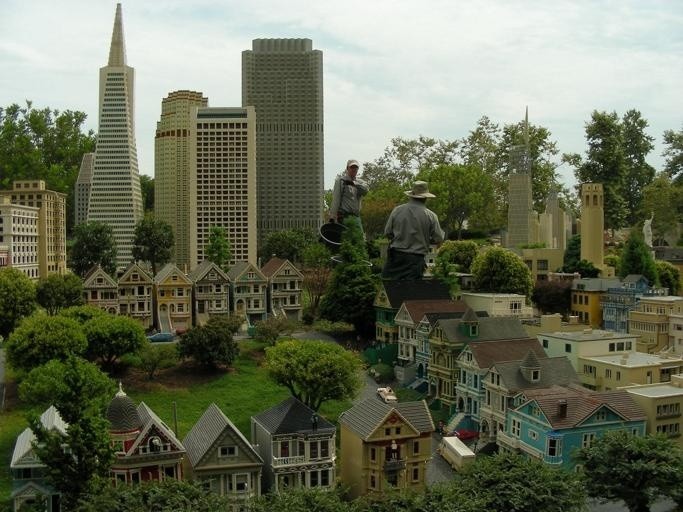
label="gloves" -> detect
[329,218,335,223]
[340,179,354,186]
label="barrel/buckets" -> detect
[320,222,351,252]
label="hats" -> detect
[404,181,436,199]
[347,159,359,168]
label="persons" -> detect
[383,180,446,281]
[642,210,656,261]
[328,159,370,233]
